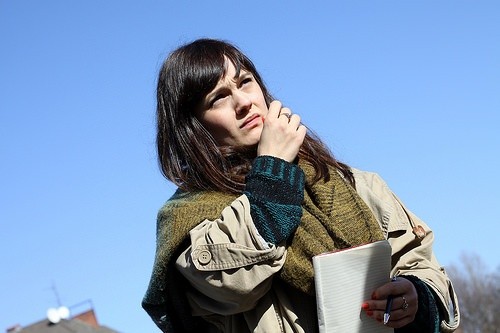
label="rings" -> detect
[279,112,291,120]
[402,296,408,309]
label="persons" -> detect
[141,38,461,333]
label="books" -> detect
[312,240,394,333]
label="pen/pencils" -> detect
[382,274,397,324]
